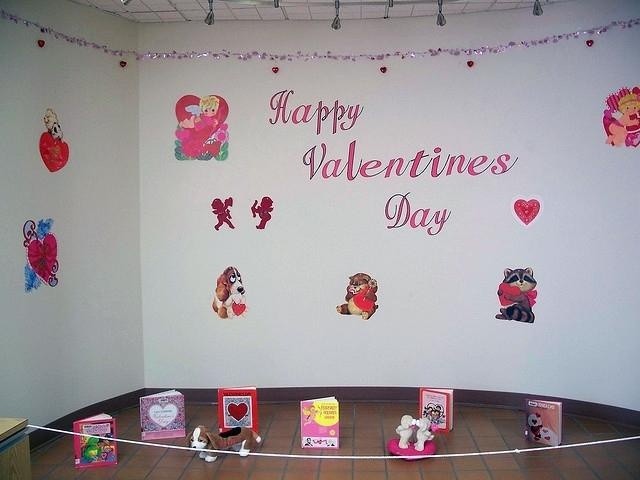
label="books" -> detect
[139,387,188,441]
[525,397,564,449]
[216,387,259,433]
[420,387,455,433]
[72,410,118,469]
[298,393,341,452]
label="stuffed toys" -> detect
[185,424,262,463]
[388,415,436,461]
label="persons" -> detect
[210,196,235,232]
[304,438,314,447]
[251,196,273,229]
